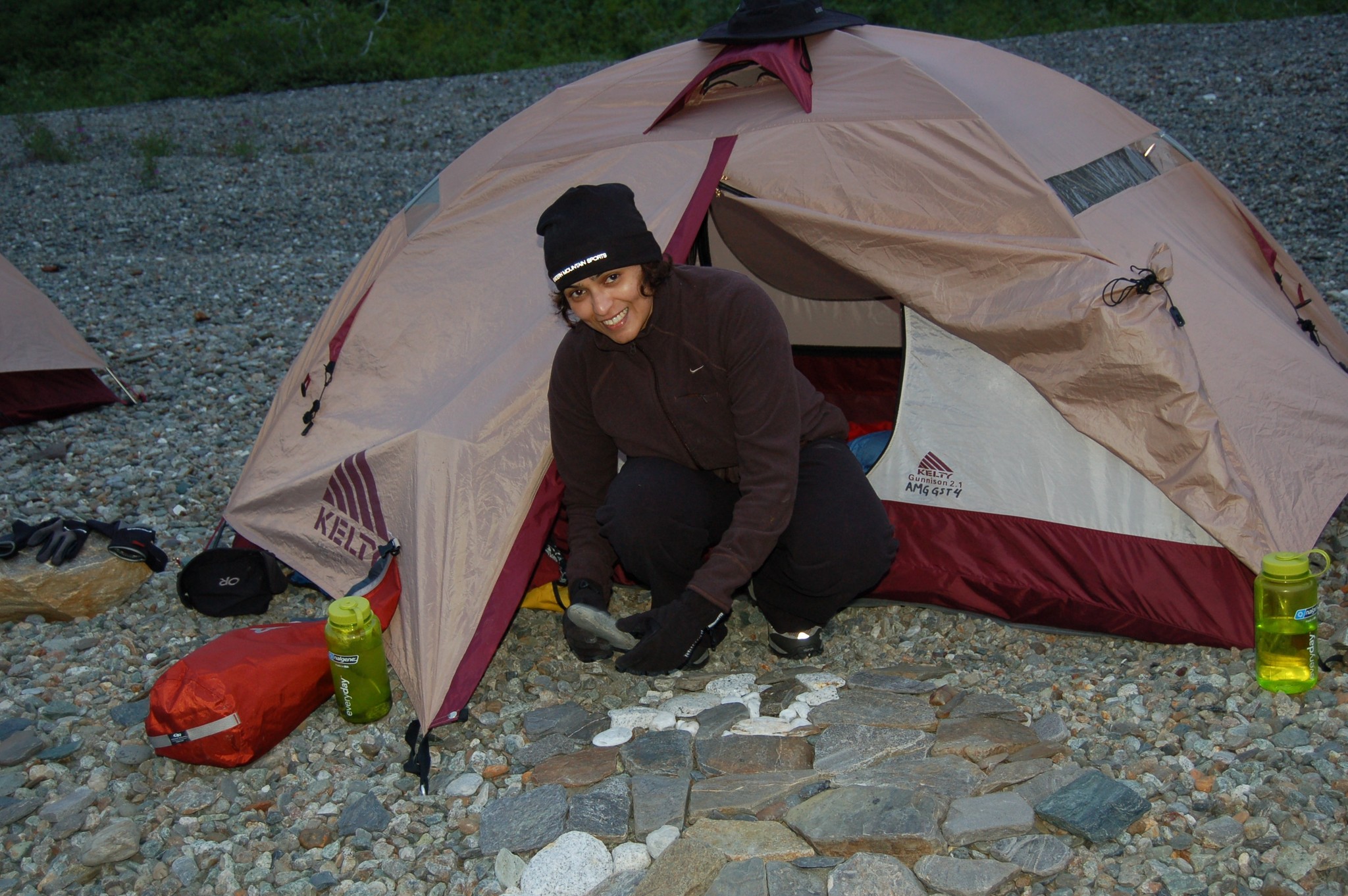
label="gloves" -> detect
[614,591,732,677]
[562,579,614,663]
[0,519,34,557]
[28,516,86,567]
[85,518,167,575]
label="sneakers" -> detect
[765,619,826,658]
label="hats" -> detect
[698,0,868,43]
[537,184,661,291]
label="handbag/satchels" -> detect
[144,622,338,766]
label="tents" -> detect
[0,251,142,422]
[198,0,1347,763]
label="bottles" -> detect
[325,596,393,725]
[1254,549,1330,694]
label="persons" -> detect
[535,183,899,677]
